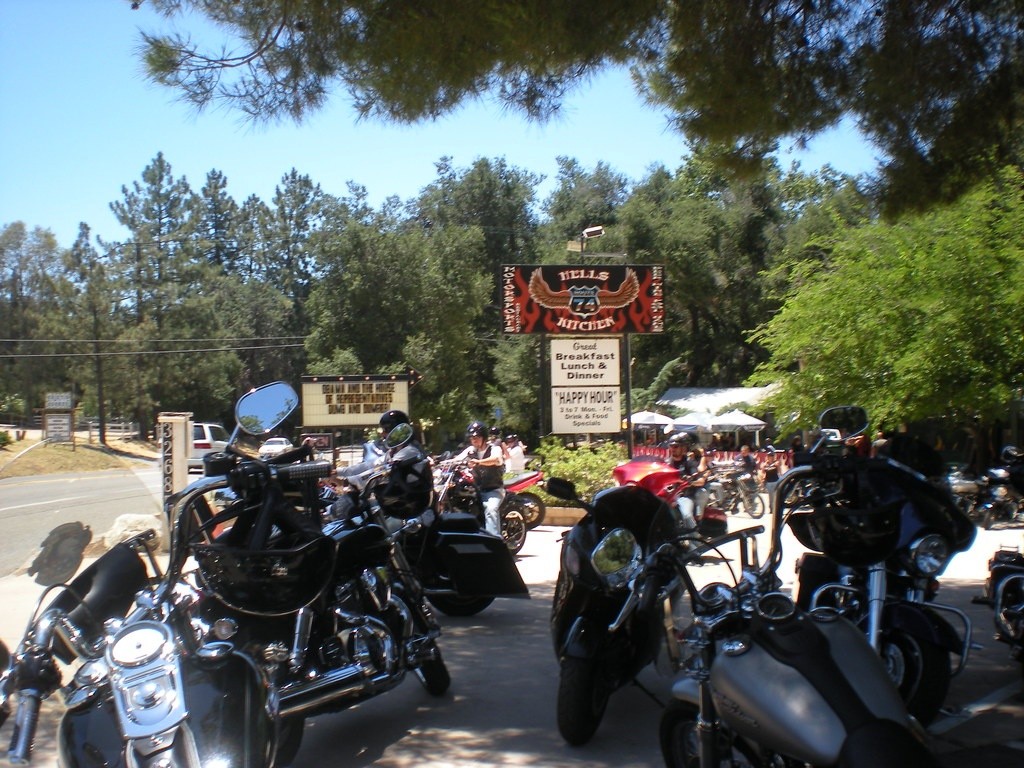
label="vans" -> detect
[188,423,233,473]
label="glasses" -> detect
[669,445,685,448]
[382,427,392,433]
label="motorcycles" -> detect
[547,445,1024,768]
[1,380,546,768]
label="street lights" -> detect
[580,226,604,265]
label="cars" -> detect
[258,438,293,458]
[110,417,130,429]
[80,417,100,429]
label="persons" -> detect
[662,430,810,549]
[869,431,888,460]
[325,409,526,549]
[302,436,321,462]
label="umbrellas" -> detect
[624,408,766,451]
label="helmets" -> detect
[490,428,500,435]
[785,497,909,567]
[373,480,430,520]
[505,435,518,442]
[191,529,337,617]
[679,432,693,443]
[669,434,684,446]
[466,422,489,436]
[379,410,409,426]
[1001,446,1017,463]
